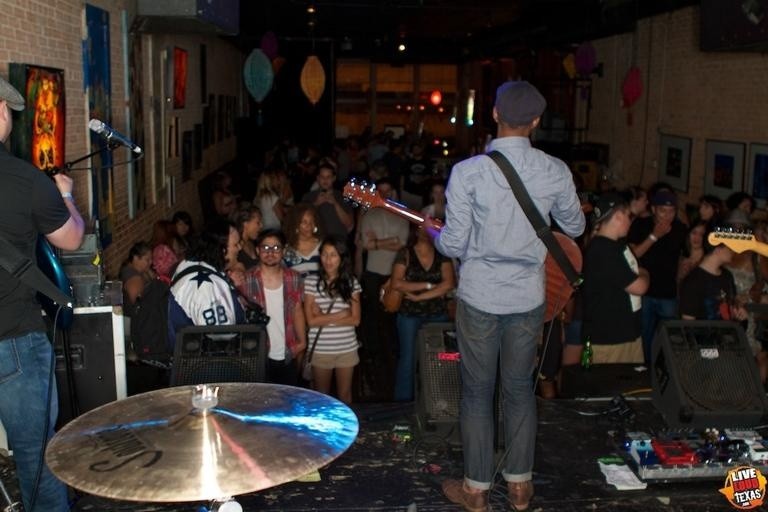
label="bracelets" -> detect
[60,189,76,199]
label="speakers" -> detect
[647,318,766,428]
[413,319,464,433]
[172,326,267,387]
[41,280,126,434]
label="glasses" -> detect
[256,244,284,254]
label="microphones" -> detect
[89,116,141,155]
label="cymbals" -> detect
[44,381,358,503]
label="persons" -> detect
[579,189,650,368]
[281,202,325,280]
[211,169,239,237]
[698,195,724,224]
[120,240,153,317]
[394,135,412,164]
[298,129,395,176]
[228,203,264,270]
[680,219,750,321]
[385,219,454,404]
[303,236,363,404]
[722,209,767,386]
[0,76,84,511]
[238,229,306,385]
[166,218,257,384]
[627,191,691,375]
[676,222,709,306]
[362,177,411,365]
[170,212,199,261]
[302,163,355,275]
[399,143,437,209]
[726,191,757,221]
[253,134,295,232]
[150,221,185,284]
[423,184,449,223]
[423,81,585,512]
[621,186,648,220]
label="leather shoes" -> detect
[505,480,534,509]
[442,476,487,511]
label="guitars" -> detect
[0,231,76,329]
[706,225,763,260]
[338,175,581,324]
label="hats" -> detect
[496,80,546,126]
[591,189,626,224]
[0,74,24,112]
[651,190,678,205]
[726,208,751,226]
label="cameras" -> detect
[244,302,271,331]
